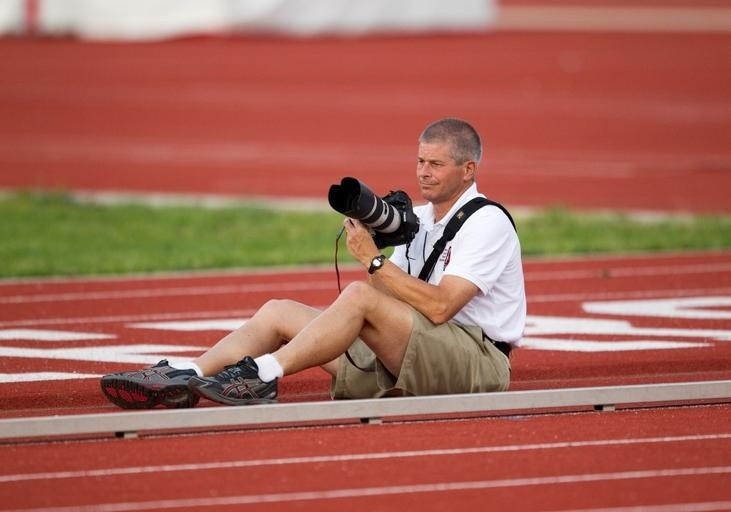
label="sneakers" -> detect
[100,359,200,407]
[188,356,278,405]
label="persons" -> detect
[100,116,528,410]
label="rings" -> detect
[346,229,350,237]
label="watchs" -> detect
[369,254,387,274]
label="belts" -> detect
[494,340,513,357]
